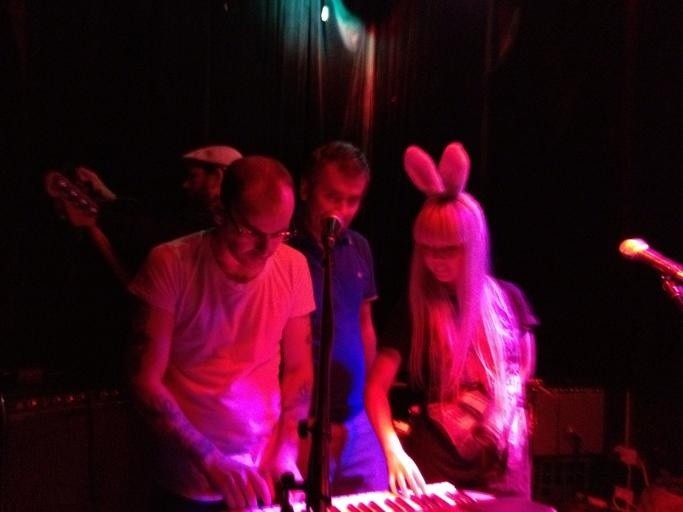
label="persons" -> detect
[363,194,536,500]
[131,154,317,511]
[181,144,242,228]
[289,145,390,495]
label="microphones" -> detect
[616,237,683,281]
[320,212,341,246]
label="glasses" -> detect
[223,209,296,243]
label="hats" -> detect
[181,145,243,167]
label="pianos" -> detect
[250,479,557,512]
